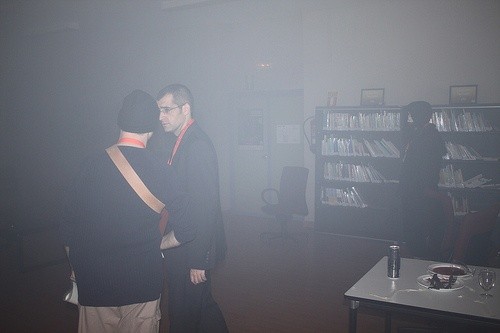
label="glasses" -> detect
[159,103,185,113]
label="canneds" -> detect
[387,246,400,279]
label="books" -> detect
[319,105,500,217]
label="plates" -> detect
[416,274,465,292]
[428,263,472,277]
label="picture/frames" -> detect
[449,84,478,104]
[326,91,338,106]
[360,87,385,107]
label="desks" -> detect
[342,255,500,333]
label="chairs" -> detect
[259,166,312,247]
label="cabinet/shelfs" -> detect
[314,103,500,259]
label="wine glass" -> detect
[478,269,496,298]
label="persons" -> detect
[400,101,450,263]
[150,86,232,333]
[60,88,194,333]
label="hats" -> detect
[120,90,159,134]
